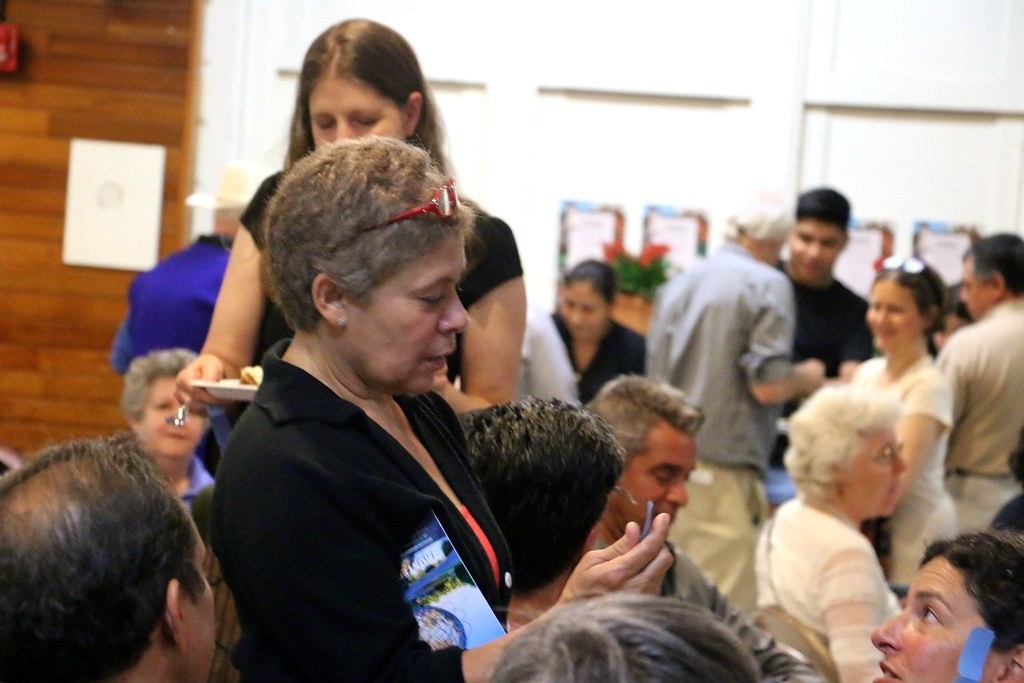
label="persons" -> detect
[1,18,1024,682]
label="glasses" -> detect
[863,442,904,464]
[881,256,944,307]
[358,176,461,234]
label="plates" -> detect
[189,378,258,401]
